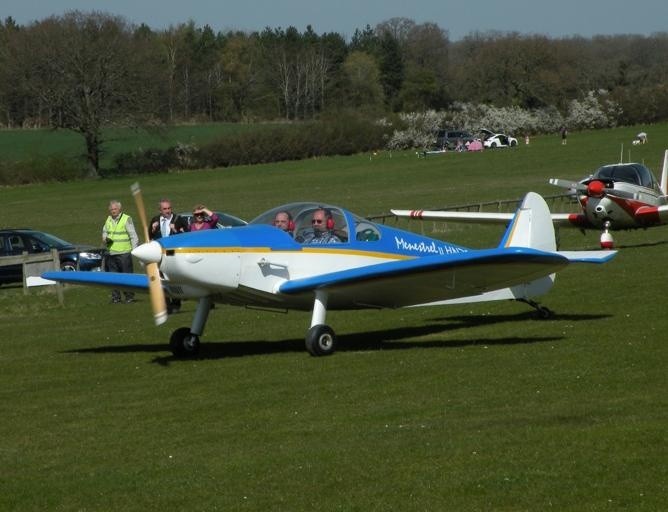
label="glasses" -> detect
[312,219,322,224]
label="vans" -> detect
[437,130,473,150]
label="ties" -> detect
[161,218,167,237]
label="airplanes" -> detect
[390,150,668,248]
[40,182,619,359]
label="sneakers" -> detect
[127,298,137,303]
[109,299,122,304]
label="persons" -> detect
[561,126,568,145]
[102,199,139,306]
[456,135,464,152]
[524,133,529,145]
[302,208,342,245]
[636,132,648,144]
[187,204,221,310]
[273,211,305,245]
[148,199,188,315]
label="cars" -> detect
[0,229,103,285]
[149,211,248,240]
[479,128,519,149]
